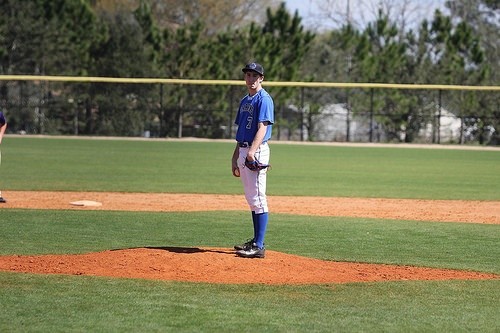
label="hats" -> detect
[242,62,265,76]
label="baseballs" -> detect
[235,169,240,176]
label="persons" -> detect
[231,61,274,258]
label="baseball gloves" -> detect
[245,156,271,171]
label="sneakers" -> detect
[234,241,254,250]
[237,244,265,258]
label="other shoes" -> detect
[0,196,5,203]
